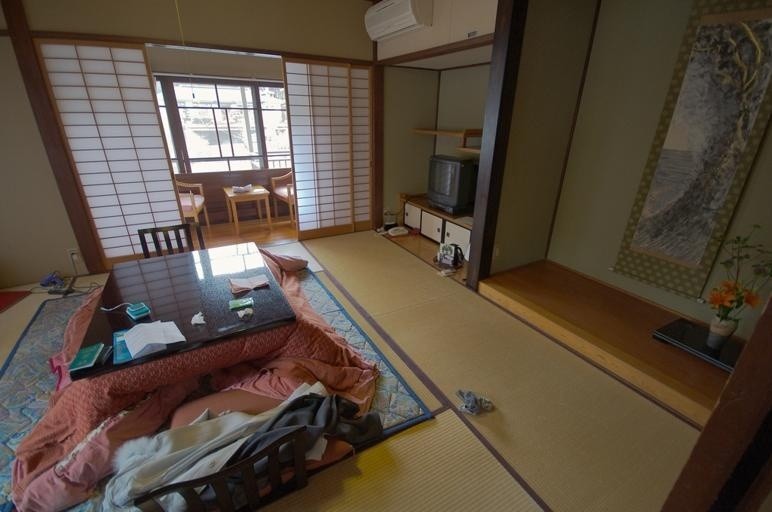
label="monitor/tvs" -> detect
[427,153,480,217]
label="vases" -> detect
[710,314,738,336]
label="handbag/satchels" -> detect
[433,242,464,269]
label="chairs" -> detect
[137,223,204,259]
[134,426,309,511]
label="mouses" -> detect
[63,289,74,295]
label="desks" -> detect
[69,241,299,392]
[222,184,273,235]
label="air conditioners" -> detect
[362,0,433,43]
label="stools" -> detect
[175,179,211,237]
[270,170,295,226]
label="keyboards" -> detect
[48,274,76,296]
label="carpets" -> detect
[0,268,437,512]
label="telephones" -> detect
[388,227,408,237]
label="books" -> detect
[67,319,167,374]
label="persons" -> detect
[165,387,285,430]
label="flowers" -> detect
[708,221,772,324]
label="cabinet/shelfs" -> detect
[401,193,475,262]
